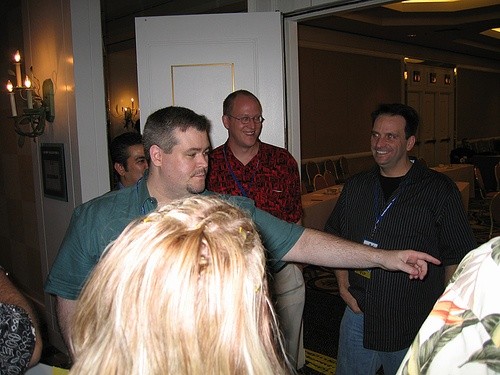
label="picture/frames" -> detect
[41,143,67,201]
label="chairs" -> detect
[306,156,348,191]
[475,162,500,239]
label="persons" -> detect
[109,130,146,192]
[202,90,310,375]
[68,199,301,375]
[50,108,441,363]
[0,266,45,375]
[393,231,500,375]
[324,103,474,375]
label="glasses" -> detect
[226,113,265,125]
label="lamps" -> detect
[124,98,140,130]
[6,50,55,144]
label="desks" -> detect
[302,164,474,233]
